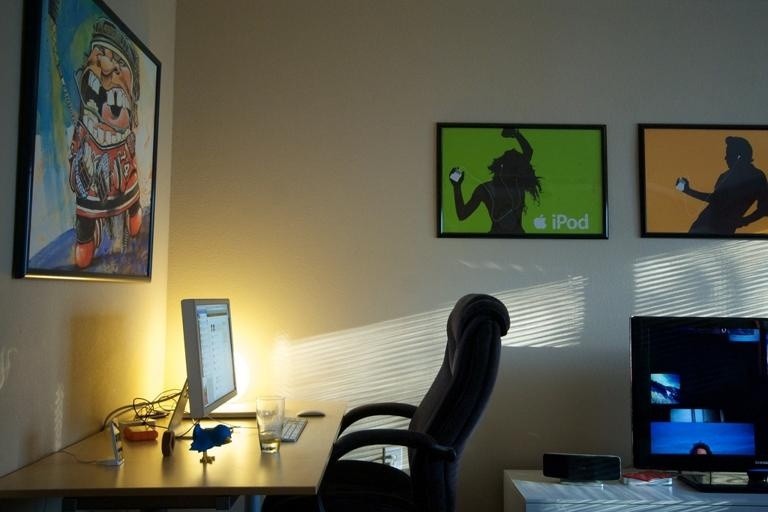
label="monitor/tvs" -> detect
[166,298,237,439]
[628,315,768,493]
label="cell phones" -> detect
[449,169,462,183]
[675,180,686,191]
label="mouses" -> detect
[297,410,326,417]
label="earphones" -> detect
[738,155,741,159]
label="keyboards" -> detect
[281,416,308,442]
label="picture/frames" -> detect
[12,0,161,283]
[638,123,768,239]
[436,122,609,239]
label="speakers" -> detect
[542,452,622,482]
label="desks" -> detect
[503,467,768,512]
[0,400,348,512]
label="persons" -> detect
[675,137,768,234]
[449,129,543,233]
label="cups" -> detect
[254,395,285,454]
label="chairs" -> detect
[260,293,510,512]
